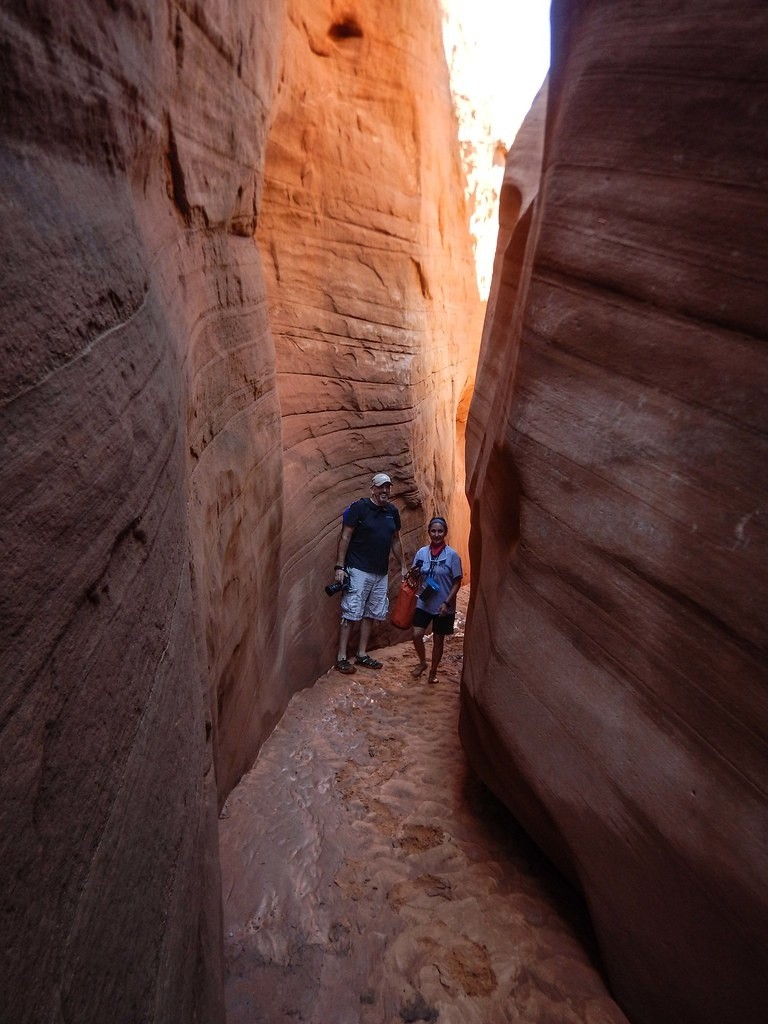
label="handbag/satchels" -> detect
[392,569,420,630]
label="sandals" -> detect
[355,655,383,669]
[335,658,357,675]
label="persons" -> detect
[408,517,463,684]
[334,474,409,674]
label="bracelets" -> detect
[334,566,344,570]
[443,601,451,608]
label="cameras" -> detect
[325,577,351,597]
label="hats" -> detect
[372,473,393,488]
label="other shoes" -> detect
[429,671,439,684]
[411,663,428,677]
